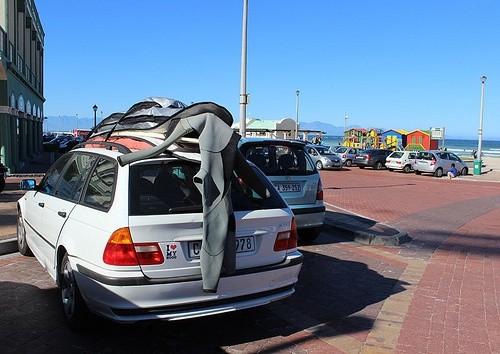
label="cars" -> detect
[306,145,343,170]
[14,130,306,327]
[42,133,85,152]
[353,148,400,170]
[236,136,327,243]
[384,151,425,173]
[323,145,366,167]
[413,150,469,177]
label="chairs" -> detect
[247,154,271,175]
[276,154,298,175]
[140,178,154,196]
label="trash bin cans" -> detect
[473,159,482,175]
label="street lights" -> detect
[477,75,487,161]
[92,104,98,129]
[295,90,300,140]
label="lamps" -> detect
[43,116,48,119]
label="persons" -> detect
[312,137,322,146]
[472,149,474,158]
[447,163,457,179]
